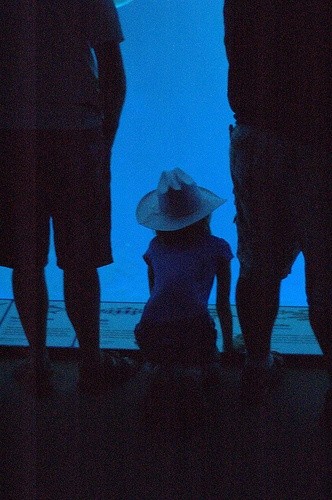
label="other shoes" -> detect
[241,351,289,400]
[14,361,54,396]
[76,352,135,391]
[318,390,332,428]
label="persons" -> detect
[1,2,128,387]
[131,169,237,401]
[220,0,332,386]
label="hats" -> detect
[136,168,225,232]
